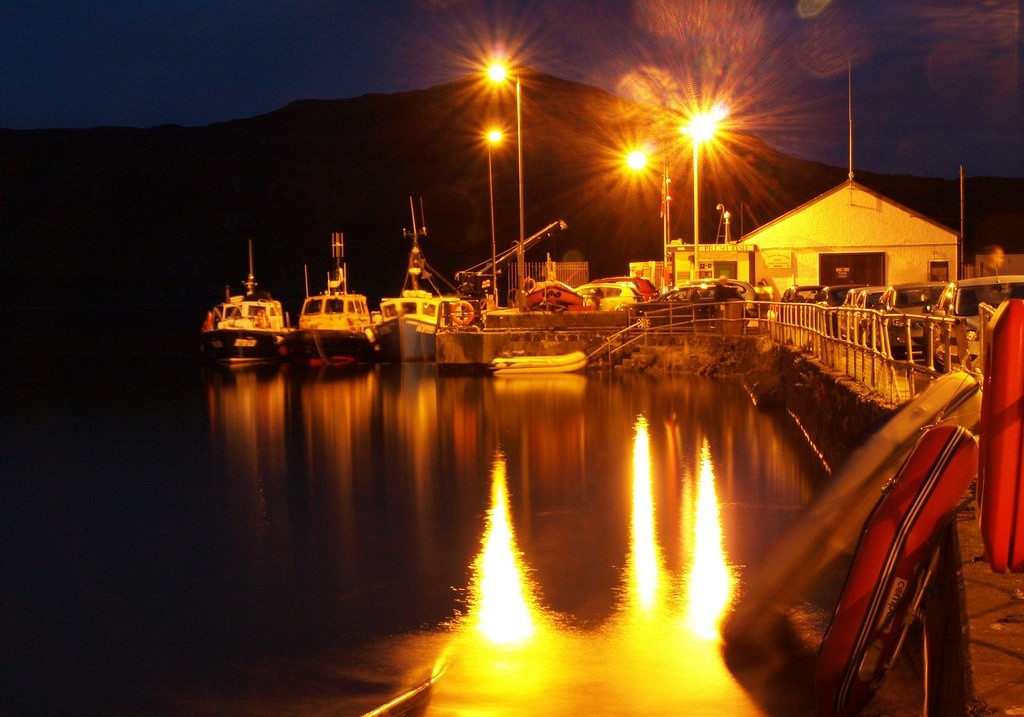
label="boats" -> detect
[199,239,297,371]
[492,352,588,374]
[525,278,584,311]
[377,195,475,363]
[297,232,379,365]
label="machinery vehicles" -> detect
[454,220,569,311]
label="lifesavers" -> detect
[257,310,269,329]
[450,301,475,326]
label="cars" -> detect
[628,279,757,332]
[922,275,1024,373]
[872,282,949,360]
[838,286,886,344]
[815,284,867,337]
[776,286,824,324]
[575,277,659,312]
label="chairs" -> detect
[798,295,805,303]
[899,293,908,305]
[1008,285,1024,300]
[960,290,978,313]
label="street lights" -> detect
[624,151,671,263]
[485,130,507,310]
[486,64,527,311]
[686,116,717,278]
